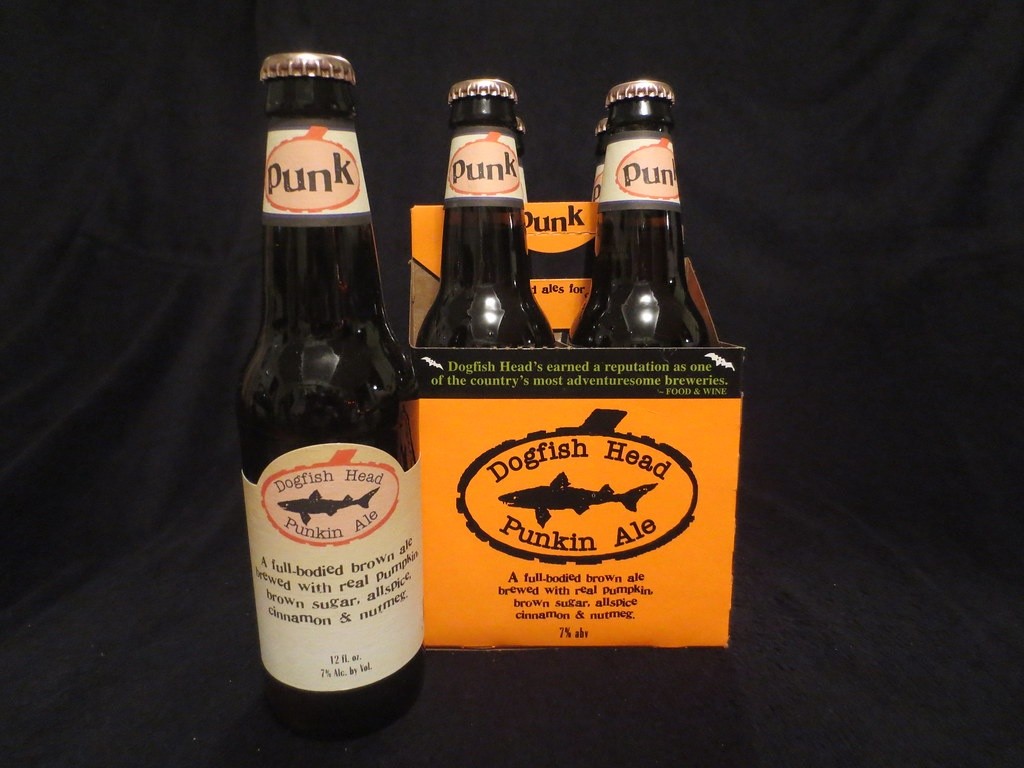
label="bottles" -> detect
[416,79,555,349]
[240,52,424,735]
[568,79,708,347]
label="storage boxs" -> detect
[403,199,747,652]
